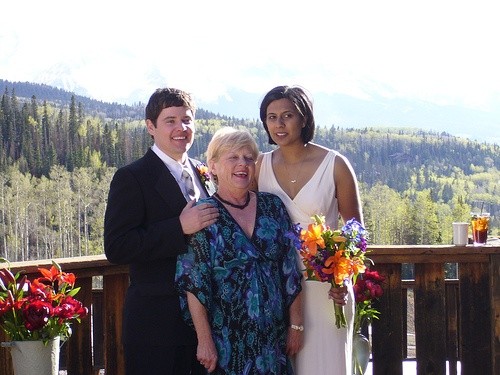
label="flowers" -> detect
[0,256,89,349]
[196,162,212,188]
[285,212,385,375]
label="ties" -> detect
[183,167,199,202]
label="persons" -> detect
[103,89,220,375]
[174,127,304,375]
[249,86,368,375]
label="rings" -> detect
[200,362,205,365]
[340,292,346,297]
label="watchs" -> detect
[289,322,305,332]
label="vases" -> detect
[1,336,61,375]
[352,328,372,375]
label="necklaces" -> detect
[288,179,297,183]
[214,191,250,210]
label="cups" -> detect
[452,223,469,246]
[472,213,489,243]
